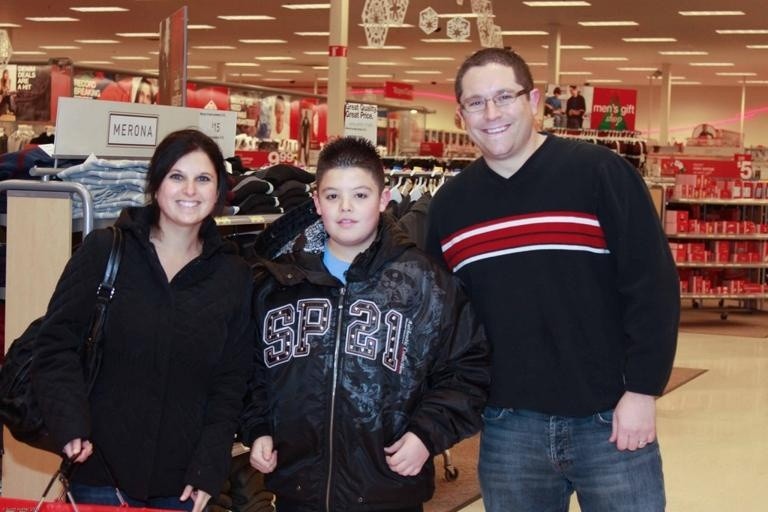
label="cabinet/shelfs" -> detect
[0,179,283,505]
[664,197,768,301]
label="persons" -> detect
[424,47,681,512]
[302,110,310,145]
[544,88,564,128]
[0,69,15,112]
[236,134,493,512]
[133,77,154,105]
[269,95,289,142]
[566,85,586,128]
[30,128,257,512]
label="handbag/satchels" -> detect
[1,225,125,456]
[0,445,187,511]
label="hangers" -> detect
[388,168,445,205]
[552,127,641,144]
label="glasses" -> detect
[461,89,528,114]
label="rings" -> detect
[639,440,648,445]
